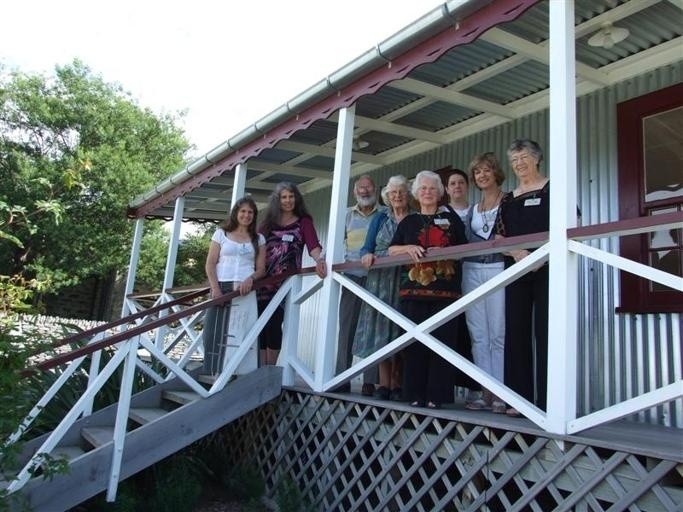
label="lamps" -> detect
[352,135,369,151]
[587,20,630,50]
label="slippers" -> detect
[465,399,492,410]
[492,401,506,414]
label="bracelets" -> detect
[314,254,325,264]
[249,274,258,282]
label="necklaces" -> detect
[480,190,502,233]
[452,200,466,209]
[420,207,443,248]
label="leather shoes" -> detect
[336,382,442,409]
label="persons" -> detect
[494,139,582,418]
[256,181,327,367]
[459,151,509,413]
[444,168,472,227]
[326,174,389,396]
[387,169,469,408]
[201,196,268,377]
[351,174,419,401]
[651,228,683,277]
